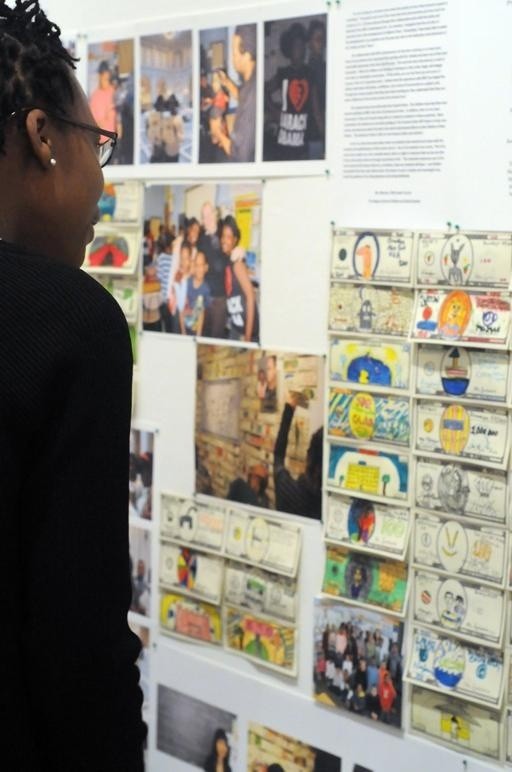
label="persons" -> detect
[129,450,153,612]
[205,729,230,772]
[0,0,149,772]
[143,203,259,341]
[317,622,399,720]
[195,356,323,519]
[90,23,326,164]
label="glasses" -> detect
[12,108,118,169]
